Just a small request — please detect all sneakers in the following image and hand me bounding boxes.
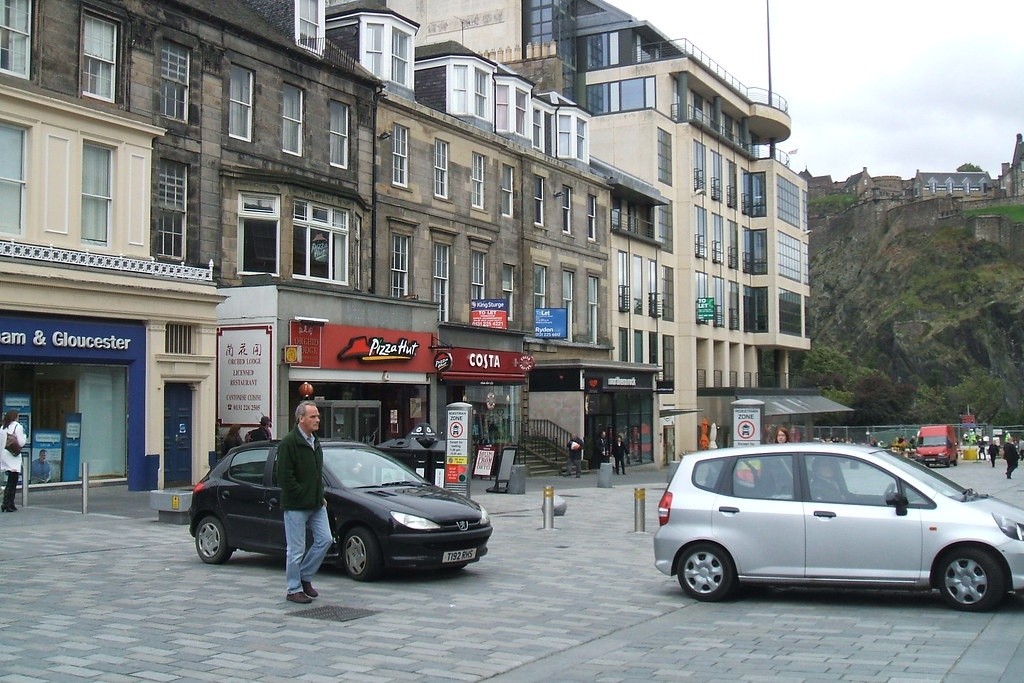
[300,579,317,597]
[286,591,312,604]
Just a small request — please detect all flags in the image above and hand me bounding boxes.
[788,149,798,154]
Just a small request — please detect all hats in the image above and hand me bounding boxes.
[259,416,269,425]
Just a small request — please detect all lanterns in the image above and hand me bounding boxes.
[299,382,314,398]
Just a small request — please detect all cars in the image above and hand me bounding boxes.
[652,443,1024,612]
[185,438,494,582]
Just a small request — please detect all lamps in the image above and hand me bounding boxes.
[552,191,565,197]
[379,131,391,140]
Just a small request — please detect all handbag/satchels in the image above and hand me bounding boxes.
[5,422,22,456]
[571,441,579,449]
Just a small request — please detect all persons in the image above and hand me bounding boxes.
[1003,437,1020,479]
[1012,438,1024,461]
[775,427,792,443]
[260,416,272,440]
[31,449,51,483]
[223,424,242,458]
[0,410,27,512]
[909,436,916,448]
[562,434,584,478]
[988,440,999,468]
[611,435,630,475]
[276,401,333,604]
[978,439,986,460]
[596,431,609,456]
[961,428,977,445]
[1004,430,1011,442]
[820,436,852,445]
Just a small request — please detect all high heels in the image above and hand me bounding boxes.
[1,503,14,512]
[10,503,18,511]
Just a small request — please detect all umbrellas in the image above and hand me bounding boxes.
[699,418,718,450]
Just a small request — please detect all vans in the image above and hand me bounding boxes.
[914,424,957,466]
